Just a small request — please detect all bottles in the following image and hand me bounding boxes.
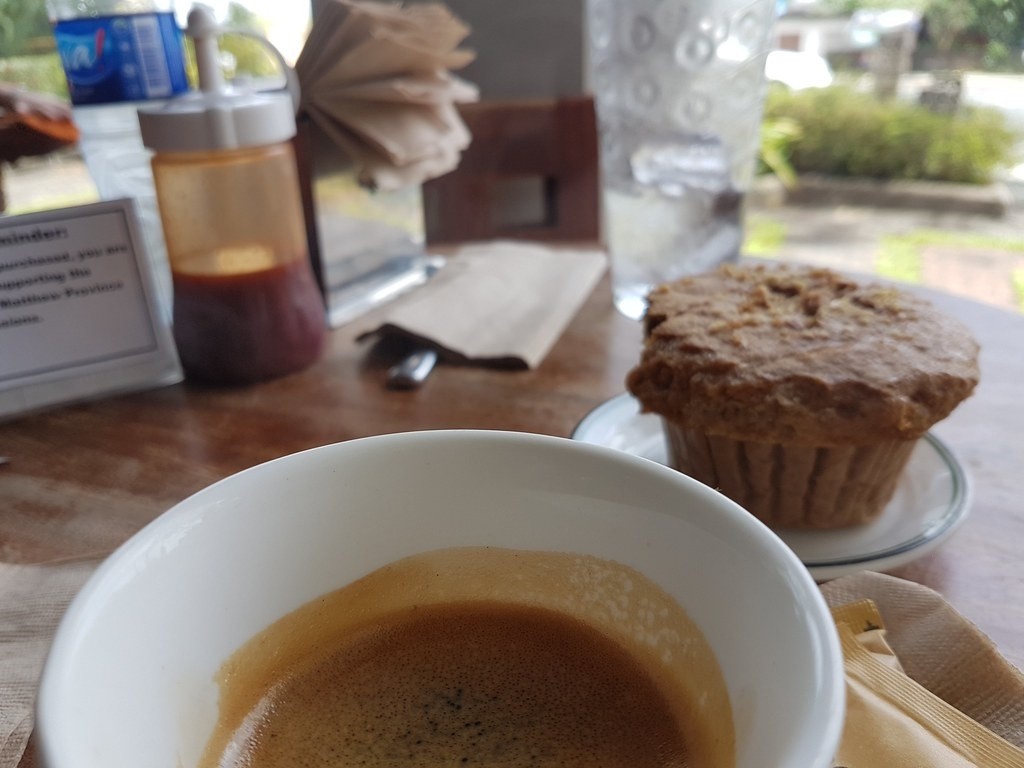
[42,0,194,329]
[136,5,329,387]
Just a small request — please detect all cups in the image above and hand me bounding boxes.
[582,0,777,320]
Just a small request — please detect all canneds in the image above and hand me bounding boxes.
[134,90,324,384]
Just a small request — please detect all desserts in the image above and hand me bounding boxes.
[626,263,983,530]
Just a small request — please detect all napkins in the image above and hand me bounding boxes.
[353,243,608,370]
[290,0,482,188]
[818,571,1024,751]
[0,560,104,768]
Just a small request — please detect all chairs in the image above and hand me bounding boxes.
[428,96,604,246]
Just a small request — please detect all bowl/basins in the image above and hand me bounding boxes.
[32,426,846,768]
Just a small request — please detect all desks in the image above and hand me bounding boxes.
[0,214,1024,768]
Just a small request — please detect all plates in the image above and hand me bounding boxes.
[570,390,971,581]
